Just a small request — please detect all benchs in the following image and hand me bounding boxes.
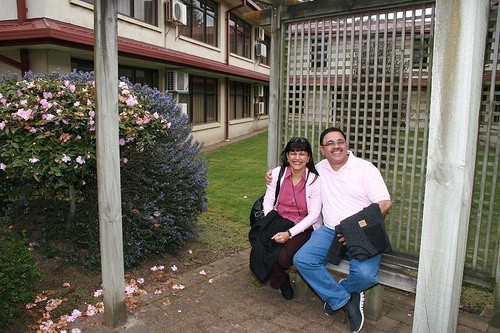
[296,251,419,321]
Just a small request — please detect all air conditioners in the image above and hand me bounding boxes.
[165,0,188,26]
[166,70,189,94]
[256,43,266,58]
[254,102,264,114]
[175,103,187,115]
[255,26,264,41]
[255,84,264,97]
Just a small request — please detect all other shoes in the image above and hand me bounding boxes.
[280,273,294,300]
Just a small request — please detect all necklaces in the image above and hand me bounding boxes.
[291,172,305,203]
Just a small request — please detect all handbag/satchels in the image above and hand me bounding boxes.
[250,195,264,227]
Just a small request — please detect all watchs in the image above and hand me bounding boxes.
[287,230,292,239]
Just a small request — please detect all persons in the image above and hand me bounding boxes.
[262,137,322,300]
[265,127,394,333]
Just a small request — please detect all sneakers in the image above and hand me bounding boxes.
[343,292,365,333]
[324,278,346,315]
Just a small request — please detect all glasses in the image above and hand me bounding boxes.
[290,137,308,144]
[323,140,346,147]
